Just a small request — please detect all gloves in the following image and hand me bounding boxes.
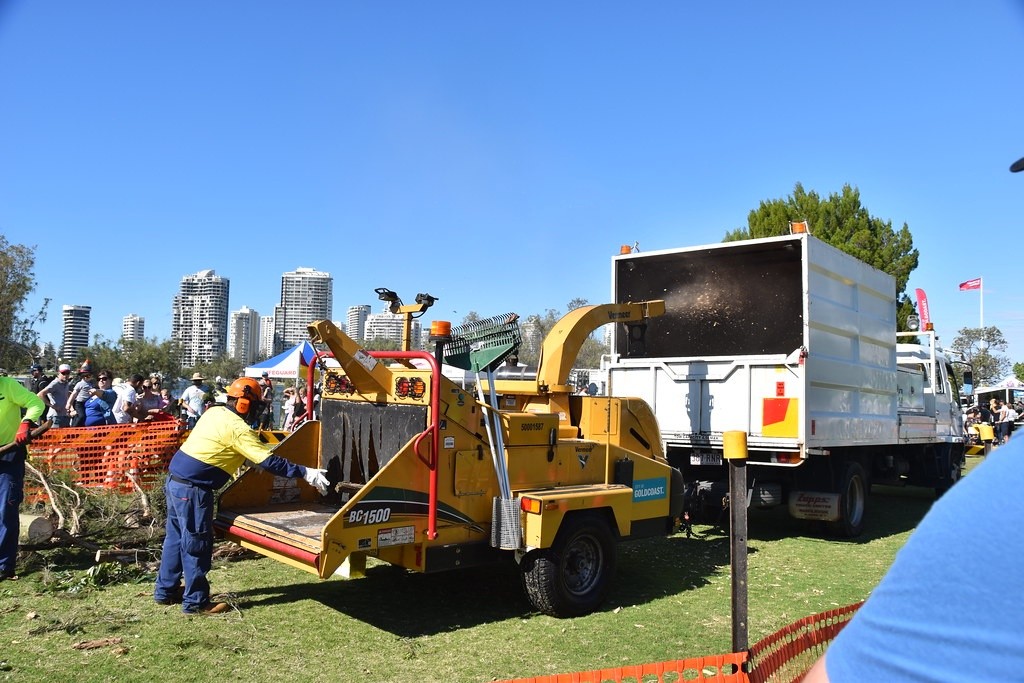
[304,466,331,497]
[14,421,30,448]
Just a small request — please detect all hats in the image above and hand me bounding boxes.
[58,364,70,373]
[189,373,207,380]
[262,372,268,376]
[28,363,43,372]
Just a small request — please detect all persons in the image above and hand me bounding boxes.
[0,359,322,493]
[963,398,1024,445]
[153,376,331,612]
[0,376,45,581]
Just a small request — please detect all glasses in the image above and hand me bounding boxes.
[142,385,152,389]
[153,382,160,385]
[61,371,70,375]
[97,377,108,381]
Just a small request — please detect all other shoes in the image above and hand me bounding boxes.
[195,602,230,614]
[0,569,15,582]
[155,597,181,605]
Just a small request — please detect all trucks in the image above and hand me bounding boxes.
[598,218,976,537]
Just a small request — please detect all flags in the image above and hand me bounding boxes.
[959,277,981,291]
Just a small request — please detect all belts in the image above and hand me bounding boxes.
[167,471,191,485]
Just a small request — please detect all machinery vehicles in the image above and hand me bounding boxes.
[214,299,688,623]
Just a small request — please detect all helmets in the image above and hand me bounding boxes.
[227,377,262,414]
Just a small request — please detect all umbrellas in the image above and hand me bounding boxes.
[244,340,323,387]
